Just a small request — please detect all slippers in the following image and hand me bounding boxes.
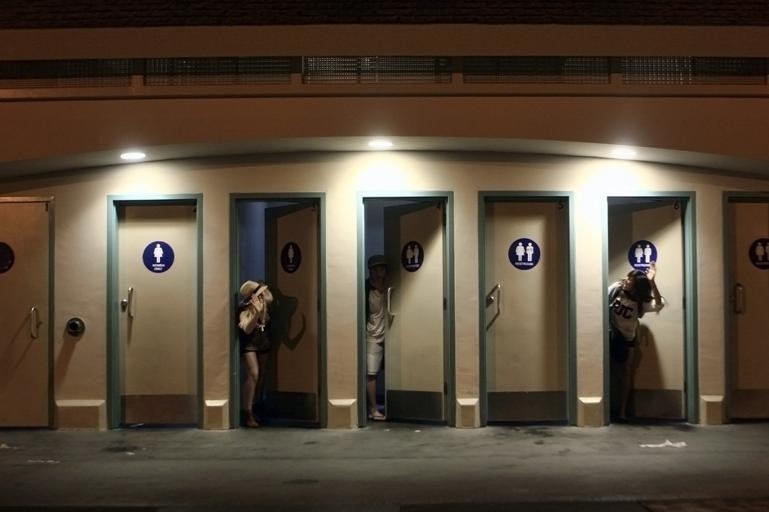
[368,413,387,420]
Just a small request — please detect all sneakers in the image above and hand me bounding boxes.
[241,410,259,427]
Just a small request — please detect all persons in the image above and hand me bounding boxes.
[236,279,273,428]
[363,253,388,423]
[608,260,666,421]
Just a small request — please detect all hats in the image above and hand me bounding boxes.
[239,279,268,305]
[365,255,392,269]
